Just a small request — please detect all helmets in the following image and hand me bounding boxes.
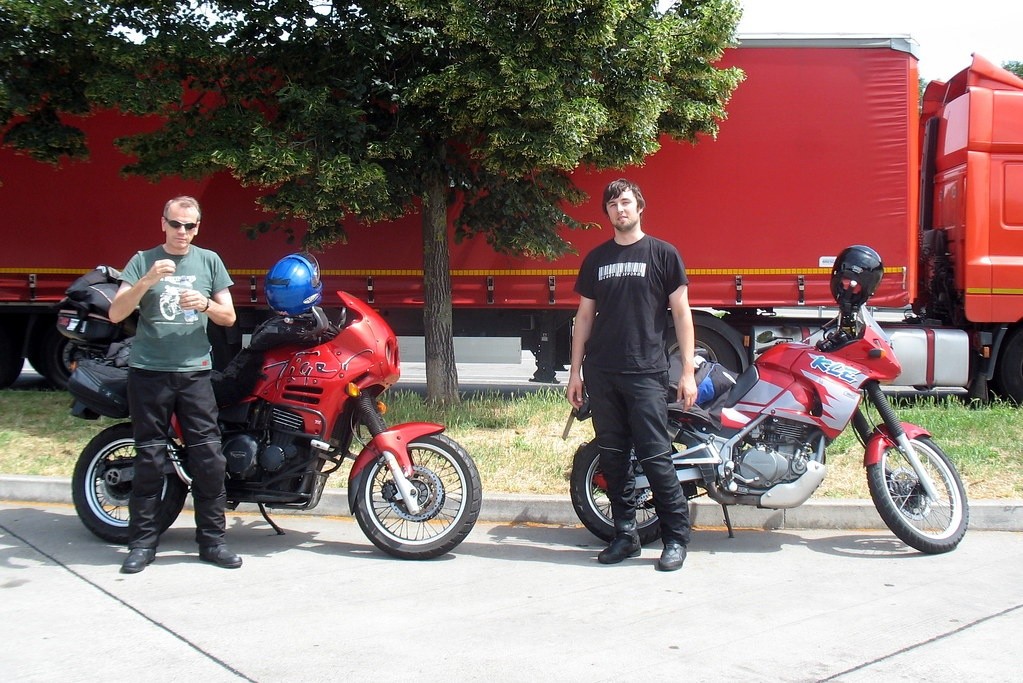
[263,250,324,318]
[831,245,884,314]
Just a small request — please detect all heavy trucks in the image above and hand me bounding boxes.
[0,31,1023,414]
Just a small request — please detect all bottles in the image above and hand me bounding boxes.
[178,275,199,323]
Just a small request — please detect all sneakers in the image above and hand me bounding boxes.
[123,548,156,573]
[199,545,243,568]
[658,539,687,571]
[598,531,641,564]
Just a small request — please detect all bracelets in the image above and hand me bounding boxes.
[200,298,210,313]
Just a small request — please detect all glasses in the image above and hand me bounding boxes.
[163,216,198,229]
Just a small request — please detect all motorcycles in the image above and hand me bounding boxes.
[562,298,969,550]
[63,287,487,560]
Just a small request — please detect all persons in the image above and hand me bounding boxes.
[107,195,245,576]
[567,177,701,569]
